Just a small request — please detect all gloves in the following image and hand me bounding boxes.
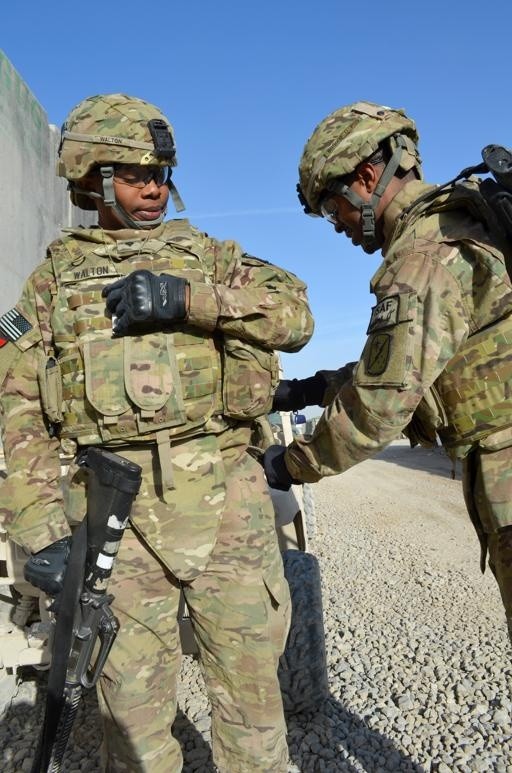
[23,537,73,595]
[259,444,303,491]
[269,374,324,414]
[102,270,186,333]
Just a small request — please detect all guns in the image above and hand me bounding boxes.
[481,144,512,231]
[17,446,142,769]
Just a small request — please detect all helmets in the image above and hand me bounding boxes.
[299,101,419,217]
[59,94,178,179]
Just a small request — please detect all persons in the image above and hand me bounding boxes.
[0,93,316,773]
[264,98,512,647]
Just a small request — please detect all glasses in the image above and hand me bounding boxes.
[321,156,385,224]
[112,164,168,187]
